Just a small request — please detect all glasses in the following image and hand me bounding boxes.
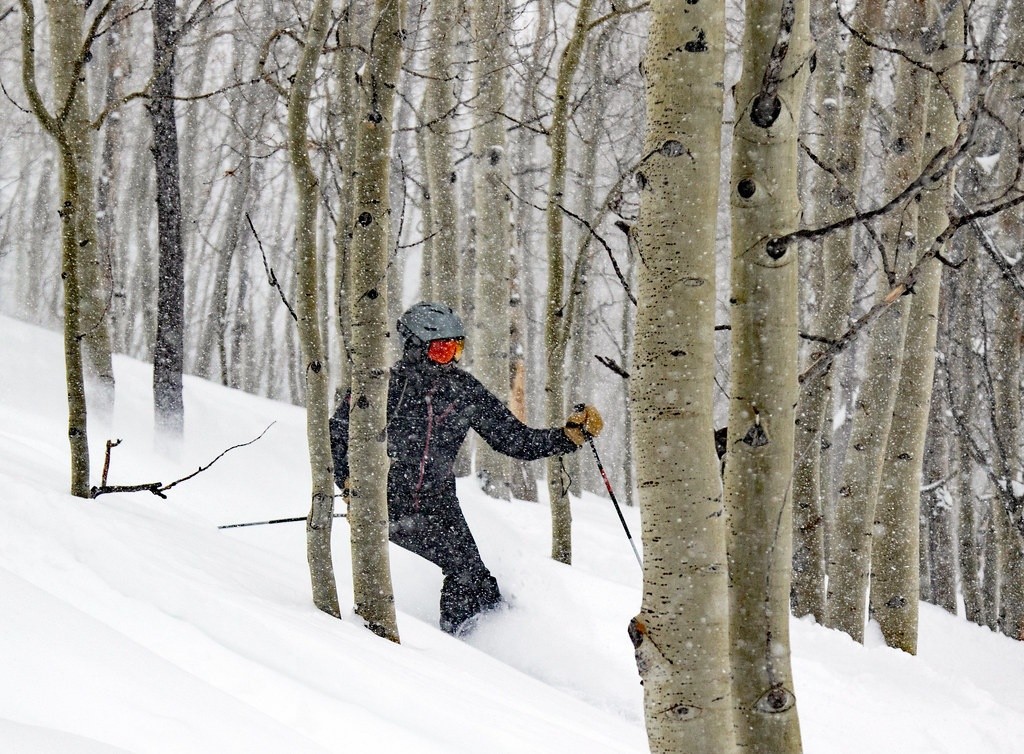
[425,335,466,364]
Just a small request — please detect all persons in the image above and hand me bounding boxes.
[328,301,604,639]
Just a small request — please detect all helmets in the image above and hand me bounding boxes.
[397,301,464,374]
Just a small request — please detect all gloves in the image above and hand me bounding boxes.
[564,404,602,446]
[342,478,350,505]
